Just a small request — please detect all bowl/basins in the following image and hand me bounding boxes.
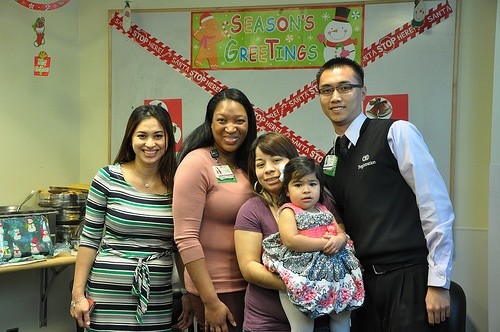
[37,194,88,223]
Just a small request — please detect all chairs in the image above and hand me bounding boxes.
[427,280,466,332]
[68,279,86,332]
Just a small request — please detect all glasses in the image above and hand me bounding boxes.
[317,83,363,97]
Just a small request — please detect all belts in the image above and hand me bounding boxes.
[361,257,429,275]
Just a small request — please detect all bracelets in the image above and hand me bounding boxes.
[180,288,188,294]
[71,295,85,308]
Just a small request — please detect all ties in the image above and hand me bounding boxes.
[336,133,350,161]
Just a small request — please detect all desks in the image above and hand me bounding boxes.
[0,252,79,331]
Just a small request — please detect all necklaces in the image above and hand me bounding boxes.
[135,162,160,188]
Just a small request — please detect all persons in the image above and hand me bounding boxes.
[69,105,194,332]
[315,56,455,332]
[171,87,257,332]
[215,167,221,174]
[233,131,346,332]
[261,156,365,332]
